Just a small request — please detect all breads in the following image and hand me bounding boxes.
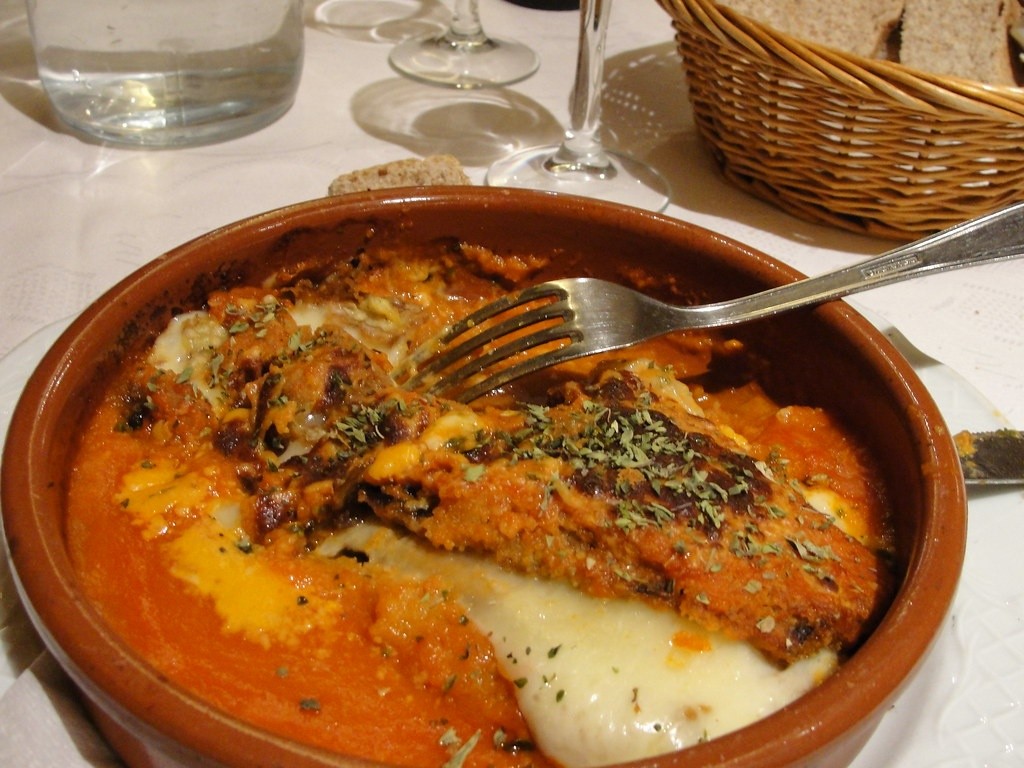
[715,0,1022,88]
[329,152,469,194]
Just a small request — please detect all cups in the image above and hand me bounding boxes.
[28,0,308,150]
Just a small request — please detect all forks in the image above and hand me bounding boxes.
[389,195,1024,405]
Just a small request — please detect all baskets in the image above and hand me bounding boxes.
[655,0,1024,241]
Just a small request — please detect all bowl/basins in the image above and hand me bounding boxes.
[2,183,968,768]
